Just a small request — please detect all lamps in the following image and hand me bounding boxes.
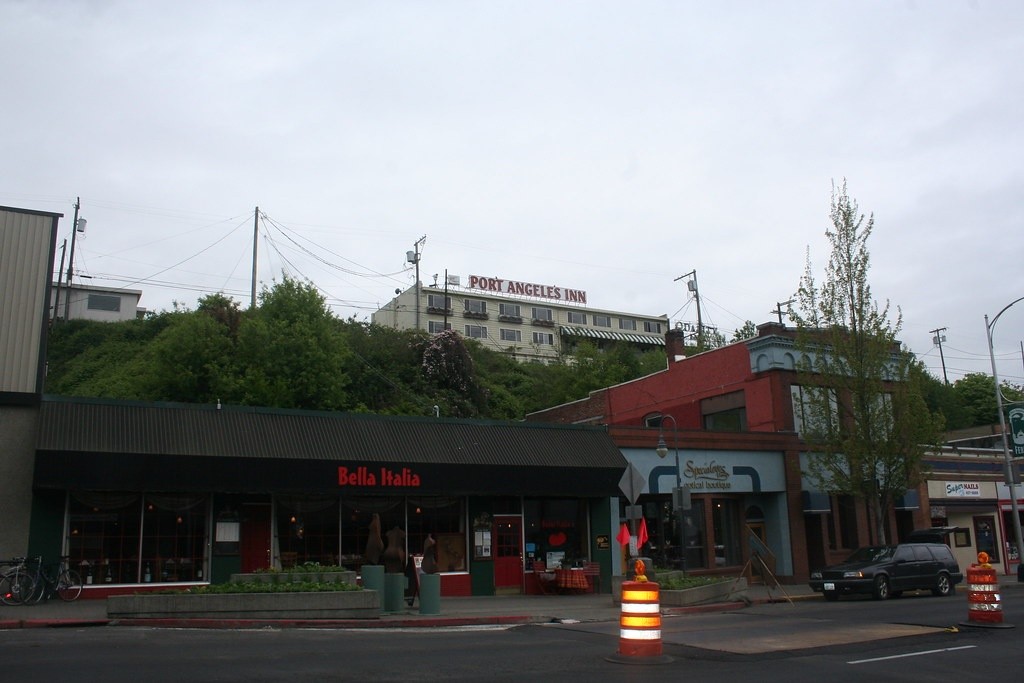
[217,399,221,409]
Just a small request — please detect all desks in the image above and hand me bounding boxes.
[556,569,589,595]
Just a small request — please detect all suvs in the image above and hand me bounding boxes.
[808,526,963,600]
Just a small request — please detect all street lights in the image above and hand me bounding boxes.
[656,414,686,578]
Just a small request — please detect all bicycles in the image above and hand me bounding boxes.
[0,554,83,606]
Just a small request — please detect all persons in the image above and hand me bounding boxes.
[80,552,111,576]
[365,513,383,555]
[384,526,406,560]
[423,534,436,574]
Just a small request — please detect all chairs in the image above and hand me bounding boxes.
[583,562,600,596]
[533,561,558,595]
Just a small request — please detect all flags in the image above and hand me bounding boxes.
[637,516,648,548]
[616,523,631,546]
